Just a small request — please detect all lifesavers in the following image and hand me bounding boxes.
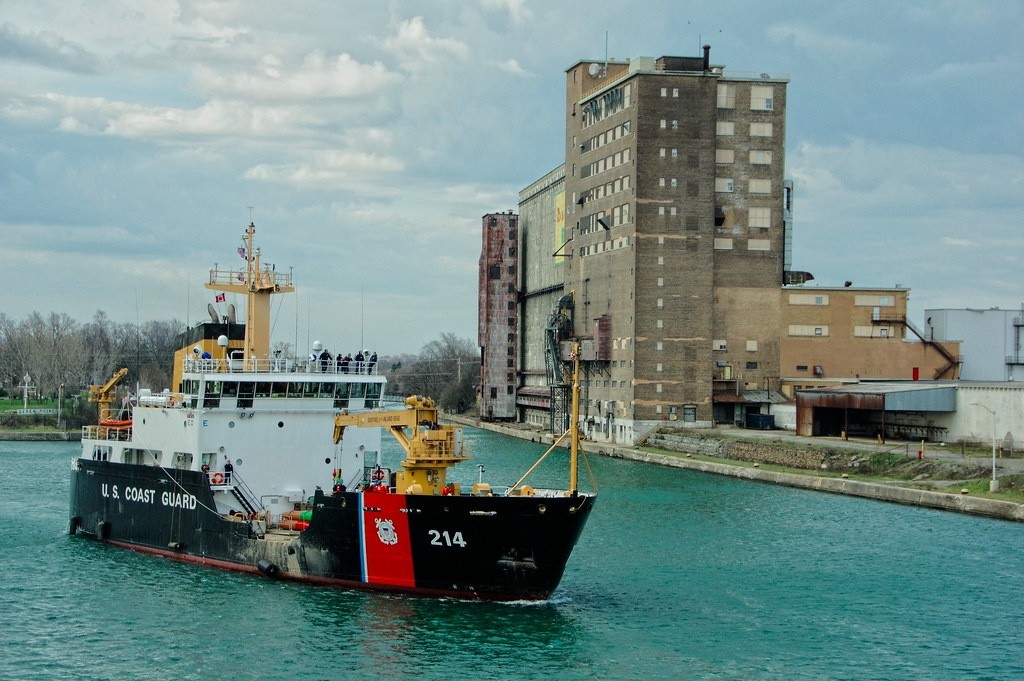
[373,469,384,481]
[167,396,175,406]
[212,473,223,484]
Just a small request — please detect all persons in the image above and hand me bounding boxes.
[201,462,211,484]
[223,460,234,485]
[368,352,377,375]
[341,353,352,374]
[336,354,343,374]
[319,349,333,373]
[355,351,365,374]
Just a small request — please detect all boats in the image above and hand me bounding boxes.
[67,221,601,605]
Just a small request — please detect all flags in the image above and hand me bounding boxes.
[216,293,226,302]
